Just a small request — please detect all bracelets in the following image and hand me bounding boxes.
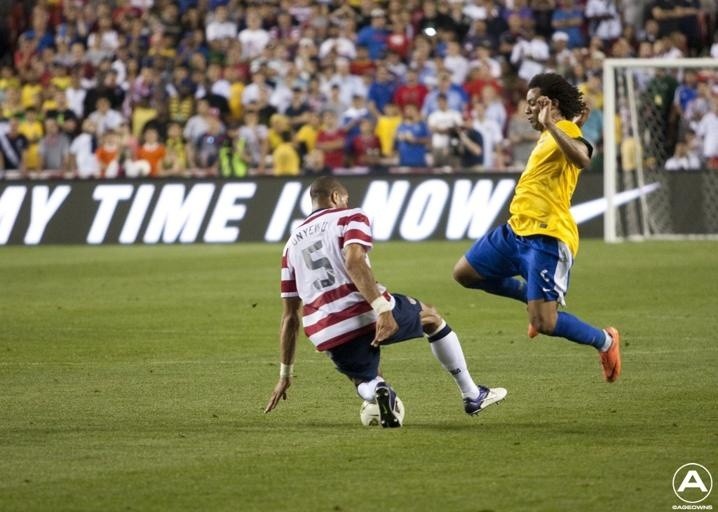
[279,362,294,379]
[369,295,393,316]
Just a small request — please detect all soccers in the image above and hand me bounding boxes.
[359,394,405,428]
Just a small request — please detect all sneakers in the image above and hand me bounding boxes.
[463,385,507,416]
[600,327,621,382]
[376,381,402,428]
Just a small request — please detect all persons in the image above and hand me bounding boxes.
[263,176,508,430]
[451,73,622,384]
